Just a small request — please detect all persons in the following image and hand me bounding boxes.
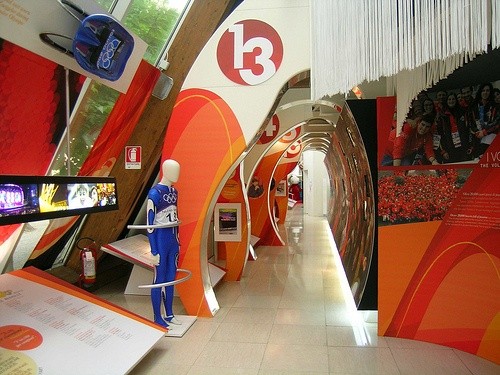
[68,184,95,210]
[381,80,500,166]
[146,159,182,330]
[247,177,264,198]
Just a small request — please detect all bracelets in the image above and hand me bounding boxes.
[431,158,436,162]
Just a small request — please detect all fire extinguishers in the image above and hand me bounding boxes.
[75,236,98,293]
